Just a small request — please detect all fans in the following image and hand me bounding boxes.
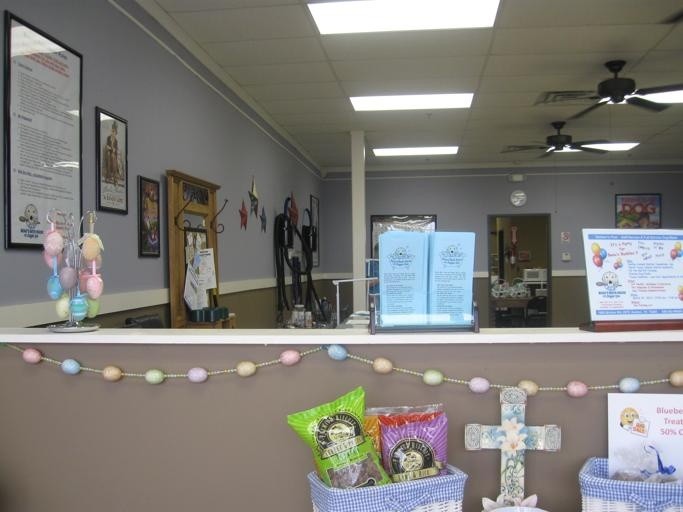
[509,121,610,159]
[567,60,683,121]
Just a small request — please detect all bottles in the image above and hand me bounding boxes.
[291,305,313,328]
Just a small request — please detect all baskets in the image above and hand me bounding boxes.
[577,456,682,512]
[306,462,469,511]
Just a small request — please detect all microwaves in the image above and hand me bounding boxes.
[523,268,548,282]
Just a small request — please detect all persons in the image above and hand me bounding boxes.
[105,121,120,188]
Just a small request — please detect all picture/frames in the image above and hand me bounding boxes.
[3,10,84,251]
[616,193,662,229]
[370,214,437,260]
[95,106,130,214]
[310,194,320,268]
[136,174,161,258]
